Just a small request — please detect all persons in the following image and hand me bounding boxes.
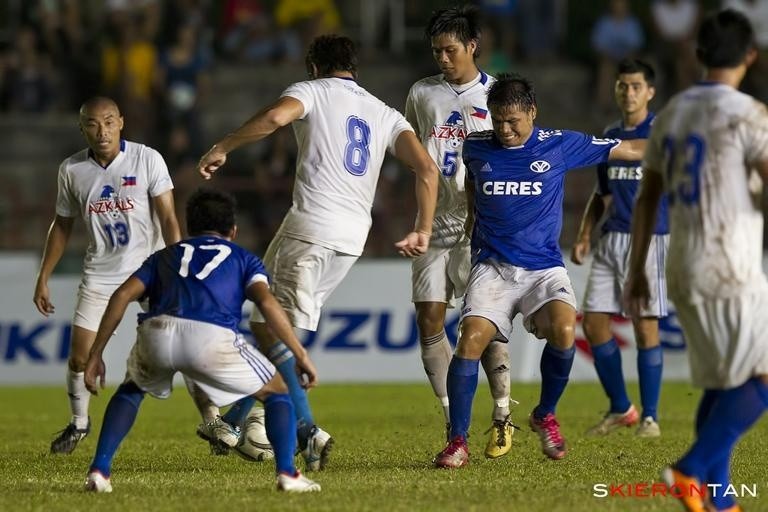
[428,67,652,474]
[0,1,768,253]
[570,55,672,442]
[82,181,321,493]
[194,31,439,475]
[401,7,522,460]
[30,94,232,456]
[614,6,768,512]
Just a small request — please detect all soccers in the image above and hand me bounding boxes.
[232,407,274,462]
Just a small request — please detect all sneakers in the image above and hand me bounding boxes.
[584,404,639,440]
[50,416,92,454]
[704,481,743,512]
[196,415,243,449]
[84,467,112,493]
[209,438,229,455]
[301,425,334,472]
[662,463,707,512]
[433,405,566,467]
[634,417,660,437]
[275,470,322,493]
